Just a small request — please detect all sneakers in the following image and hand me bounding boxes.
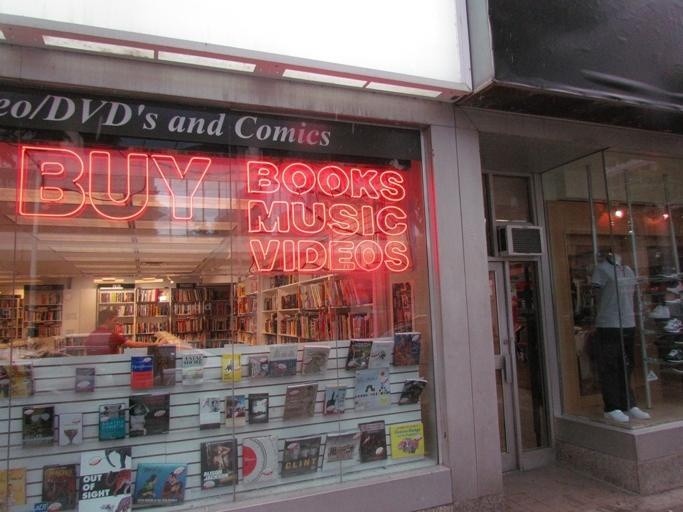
[602,409,630,423]
[623,406,650,419]
[647,272,683,373]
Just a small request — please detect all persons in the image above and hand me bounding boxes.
[591,251,651,425]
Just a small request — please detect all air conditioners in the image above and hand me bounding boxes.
[497,223,544,257]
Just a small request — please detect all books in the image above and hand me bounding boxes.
[95,283,228,348]
[182,346,356,490]
[345,332,429,463]
[229,265,377,346]
[1,345,187,511]
[0,290,63,341]
